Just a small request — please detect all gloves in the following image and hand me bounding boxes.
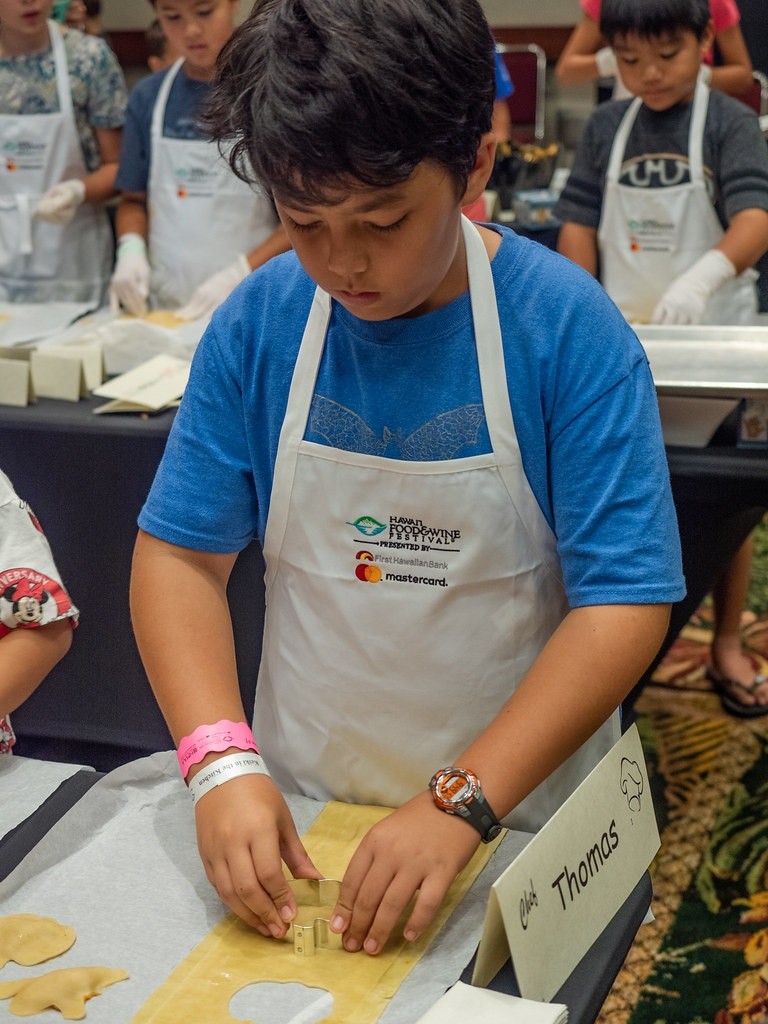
[36,180,85,226]
[653,249,737,326]
[181,253,253,319]
[109,233,150,316]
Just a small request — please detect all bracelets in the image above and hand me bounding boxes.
[177,719,264,786]
[189,752,272,809]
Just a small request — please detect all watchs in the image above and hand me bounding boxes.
[429,767,502,845]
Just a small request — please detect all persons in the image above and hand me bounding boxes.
[131,0,688,956]
[556,0,754,101]
[51,0,174,70]
[0,470,78,757]
[553,1,768,718]
[0,0,130,303]
[114,0,292,316]
[491,55,515,143]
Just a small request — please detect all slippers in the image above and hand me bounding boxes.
[706,666,768,714]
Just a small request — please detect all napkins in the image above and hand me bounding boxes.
[414,980,569,1024]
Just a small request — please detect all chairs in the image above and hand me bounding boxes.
[494,43,547,145]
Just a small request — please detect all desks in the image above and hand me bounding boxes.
[0,300,768,772]
[494,187,600,284]
[0,751,653,1024]
